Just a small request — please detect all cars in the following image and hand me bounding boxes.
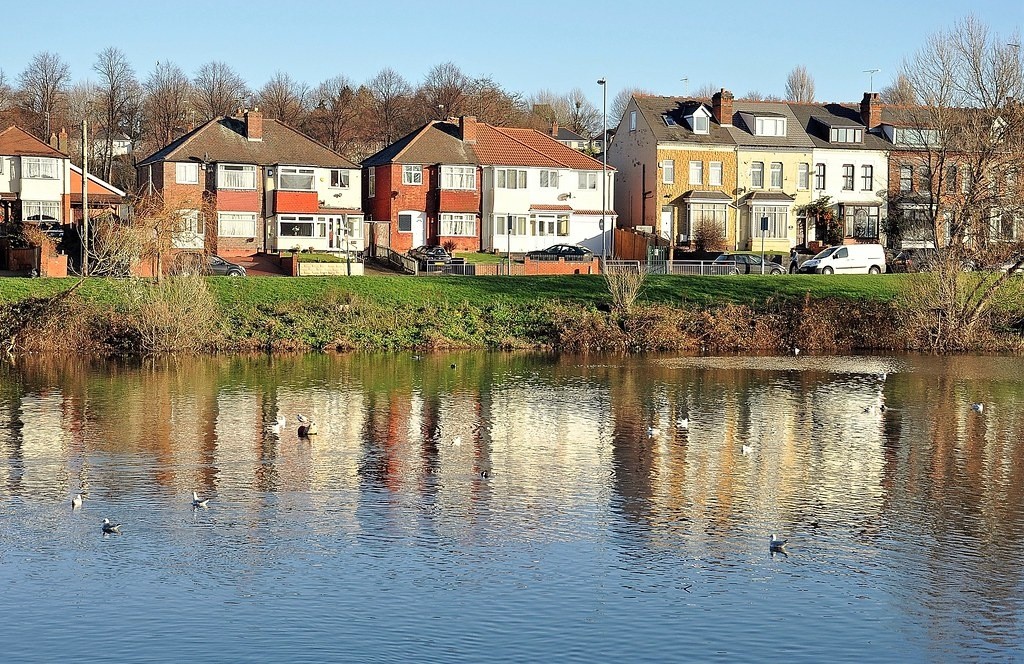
[523,244,594,263]
[711,253,787,276]
[409,245,452,271]
[984,250,1024,272]
[890,249,975,274]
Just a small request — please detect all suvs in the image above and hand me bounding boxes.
[168,250,247,277]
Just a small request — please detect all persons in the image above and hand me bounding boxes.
[789,247,799,274]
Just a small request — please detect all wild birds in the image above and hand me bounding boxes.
[859,403,886,413]
[101,517,122,533]
[191,491,211,509]
[768,531,790,547]
[293,411,317,442]
[450,363,458,369]
[646,416,689,437]
[479,466,494,478]
[740,443,753,455]
[793,346,798,352]
[973,400,983,412]
[451,437,461,446]
[8,419,24,425]
[410,354,423,360]
[87,411,101,422]
[72,493,84,509]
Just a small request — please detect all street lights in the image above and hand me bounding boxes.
[597,76,607,261]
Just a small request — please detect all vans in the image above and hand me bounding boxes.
[798,244,886,275]
